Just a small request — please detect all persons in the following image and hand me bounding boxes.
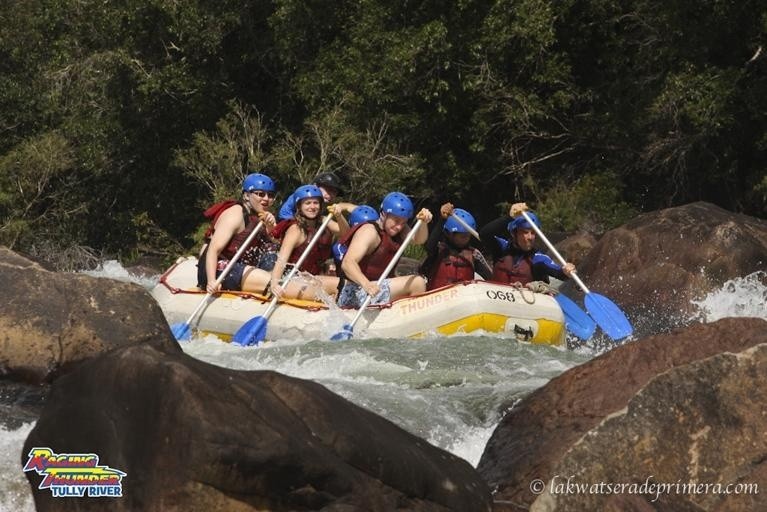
[267,183,348,306]
[332,201,380,275]
[478,203,578,295]
[337,189,432,307]
[421,200,492,294]
[196,170,280,294]
[278,171,342,222]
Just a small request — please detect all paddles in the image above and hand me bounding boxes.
[516,202,632,342]
[230,210,328,343]
[167,222,266,341]
[447,208,597,341]
[331,215,423,336]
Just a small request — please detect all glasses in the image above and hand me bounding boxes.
[251,190,275,198]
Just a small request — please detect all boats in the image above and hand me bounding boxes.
[153,254,567,352]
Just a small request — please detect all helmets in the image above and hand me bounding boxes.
[508,212,539,231]
[313,174,344,194]
[383,193,413,218]
[243,174,275,192]
[348,206,378,224]
[294,185,324,204]
[444,209,476,233]
